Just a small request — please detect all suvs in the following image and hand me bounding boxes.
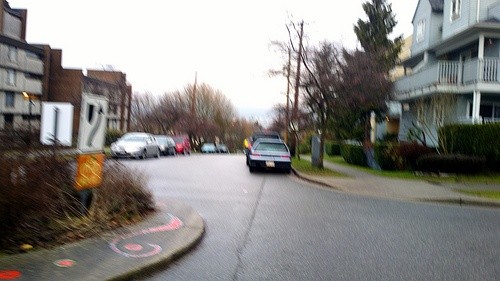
[246,134,280,167]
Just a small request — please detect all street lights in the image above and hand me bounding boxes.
[21,90,35,130]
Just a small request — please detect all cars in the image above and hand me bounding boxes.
[110,132,160,159]
[248,138,292,174]
[154,135,175,156]
[201,142,216,154]
[170,136,191,155]
[218,144,228,153]
